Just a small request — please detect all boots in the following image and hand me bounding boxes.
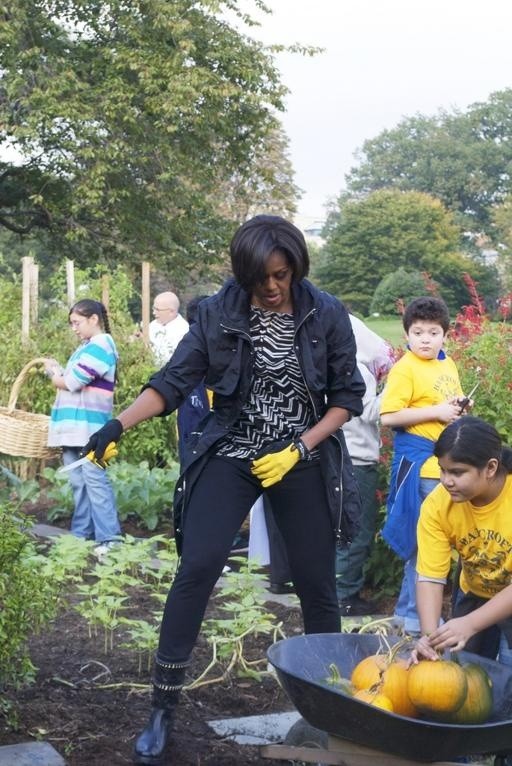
[133,652,191,759]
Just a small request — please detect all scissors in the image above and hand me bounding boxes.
[456,380,483,416]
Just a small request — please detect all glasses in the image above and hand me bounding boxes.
[152,306,170,313]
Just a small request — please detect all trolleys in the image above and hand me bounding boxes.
[252,631,512,766]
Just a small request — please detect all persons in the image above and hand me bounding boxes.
[41,301,124,544]
[378,297,475,638]
[405,417,512,671]
[81,218,366,766]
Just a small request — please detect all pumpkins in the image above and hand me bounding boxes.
[323,654,493,724]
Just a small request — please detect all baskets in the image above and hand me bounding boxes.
[0,355,67,461]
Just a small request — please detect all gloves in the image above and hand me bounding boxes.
[249,437,311,488]
[78,418,123,469]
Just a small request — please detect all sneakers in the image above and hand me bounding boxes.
[94,540,380,617]
